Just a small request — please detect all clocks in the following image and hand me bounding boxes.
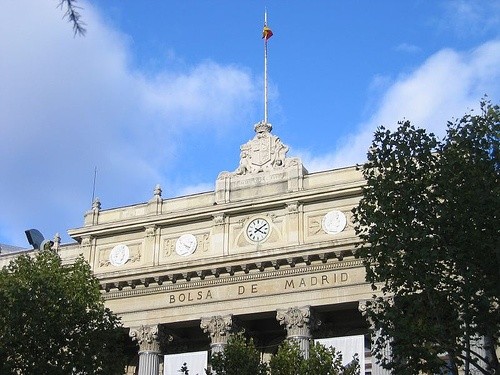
[246,218,269,242]
